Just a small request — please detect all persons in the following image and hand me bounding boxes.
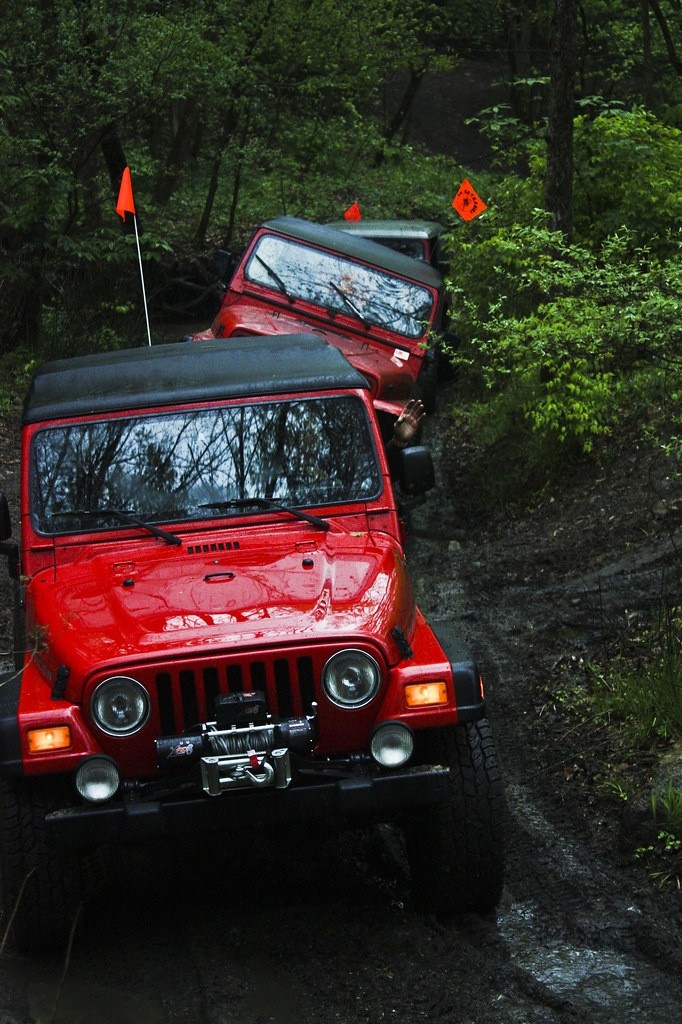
[385,398,427,472]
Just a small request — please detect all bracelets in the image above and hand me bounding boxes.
[391,438,408,447]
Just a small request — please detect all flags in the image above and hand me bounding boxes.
[451,179,488,222]
[343,201,362,223]
[116,167,135,223]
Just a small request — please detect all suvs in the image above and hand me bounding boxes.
[323,220,451,280]
[191,216,446,453]
[0,331,506,966]
